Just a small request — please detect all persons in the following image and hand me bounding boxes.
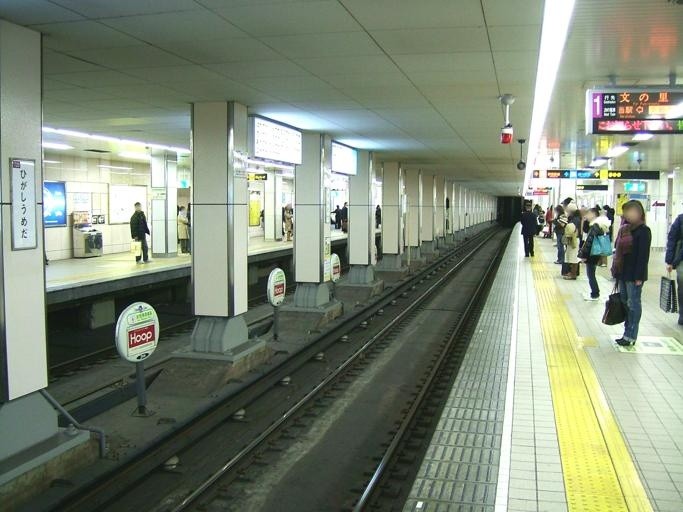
[610,199,651,347]
[282,207,293,242]
[177,202,191,253]
[130,202,153,264]
[665,213,683,325]
[521,198,615,302]
[375,205,381,228]
[341,202,348,231]
[330,205,342,229]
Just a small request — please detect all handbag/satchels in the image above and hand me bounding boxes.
[590,225,612,256]
[602,277,625,325]
[130,238,142,256]
[659,270,677,313]
[542,225,550,235]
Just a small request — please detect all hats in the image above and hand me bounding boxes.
[524,203,531,208]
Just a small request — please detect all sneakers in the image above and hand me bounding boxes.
[553,260,576,279]
[582,291,598,300]
[615,338,635,346]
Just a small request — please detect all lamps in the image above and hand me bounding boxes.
[517,139,526,171]
[501,93,516,145]
